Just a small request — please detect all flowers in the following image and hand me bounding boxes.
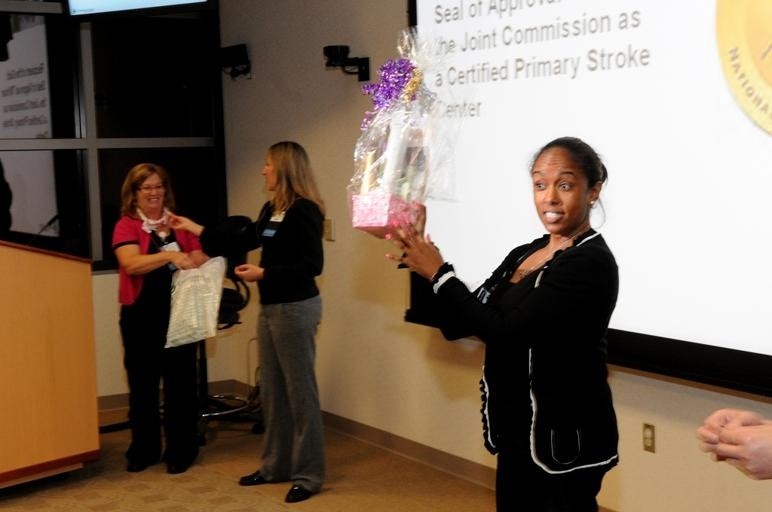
[355,55,427,133]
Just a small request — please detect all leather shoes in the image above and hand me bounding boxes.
[285,484,319,503]
[238,469,287,486]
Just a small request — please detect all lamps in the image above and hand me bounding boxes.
[218,42,254,84]
[323,44,372,83]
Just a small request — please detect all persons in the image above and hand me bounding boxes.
[385,135,623,512]
[165,140,326,507]
[112,160,212,476]
[691,407,771,484]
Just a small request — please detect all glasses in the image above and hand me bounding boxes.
[135,185,163,192]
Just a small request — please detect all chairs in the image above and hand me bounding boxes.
[160,214,254,447]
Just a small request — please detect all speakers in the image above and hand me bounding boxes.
[220,43,250,78]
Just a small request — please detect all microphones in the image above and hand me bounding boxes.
[38,214,59,235]
